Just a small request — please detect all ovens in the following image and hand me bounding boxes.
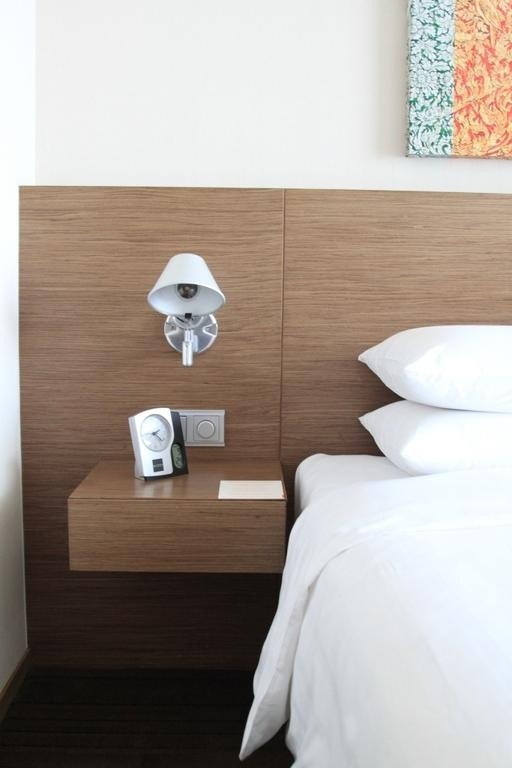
[273,453,512,767]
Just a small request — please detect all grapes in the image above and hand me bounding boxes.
[68,498,290,575]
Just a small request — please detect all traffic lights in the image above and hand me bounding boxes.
[353,326,512,477]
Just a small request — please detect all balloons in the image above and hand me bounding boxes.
[127,407,189,481]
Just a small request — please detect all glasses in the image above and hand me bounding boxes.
[146,252,227,368]
